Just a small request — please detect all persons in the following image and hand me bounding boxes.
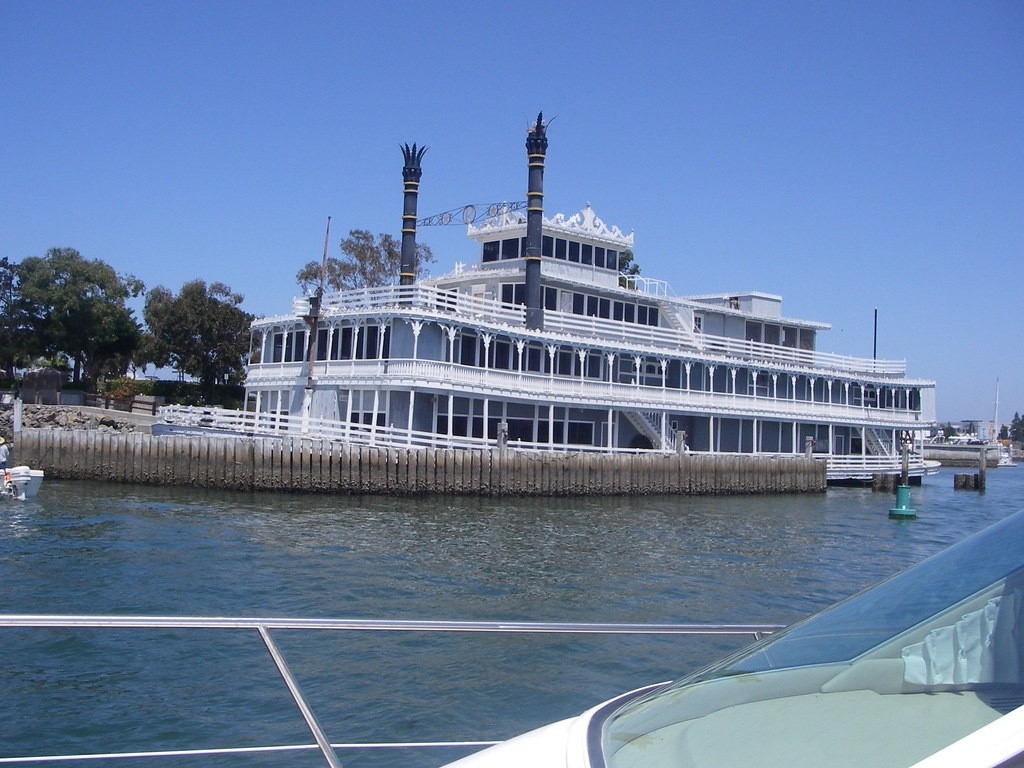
[0,437,9,469]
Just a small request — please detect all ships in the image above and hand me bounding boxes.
[161,106,948,487]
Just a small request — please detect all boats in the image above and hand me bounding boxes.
[998,442,1019,468]
[0,465,46,499]
